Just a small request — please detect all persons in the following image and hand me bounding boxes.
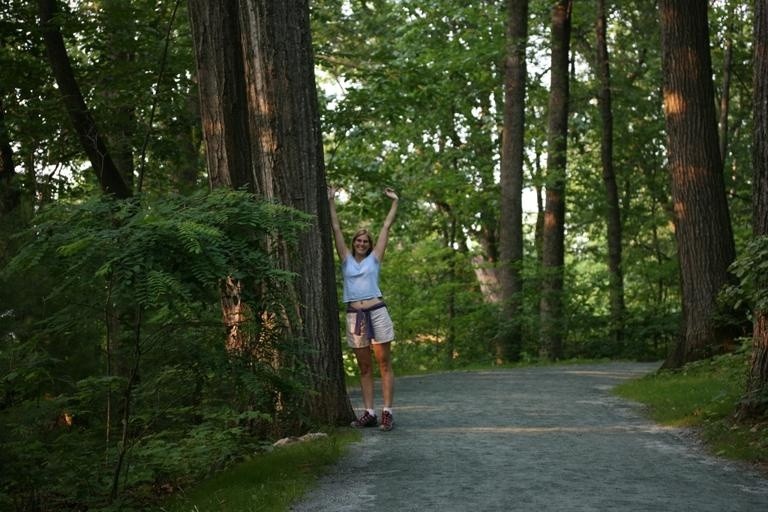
[327,184,401,431]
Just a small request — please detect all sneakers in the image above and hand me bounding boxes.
[351,410,379,429]
[380,411,394,432]
